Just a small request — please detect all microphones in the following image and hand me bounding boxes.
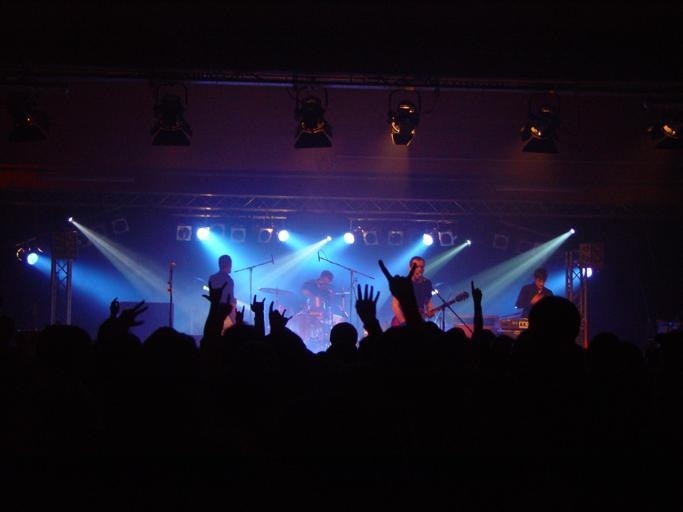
[318,251,320,261]
[272,256,274,264]
[168,257,176,267]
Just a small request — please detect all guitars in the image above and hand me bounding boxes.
[391,292,469,327]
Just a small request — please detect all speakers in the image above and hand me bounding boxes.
[64,231,79,259]
[579,242,592,268]
[591,240,610,271]
[50,232,65,259]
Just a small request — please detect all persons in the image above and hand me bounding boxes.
[1,256,681,512]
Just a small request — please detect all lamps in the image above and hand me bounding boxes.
[645,105,682,150]
[520,91,559,155]
[6,87,53,143]
[293,84,334,148]
[148,79,193,148]
[386,88,422,147]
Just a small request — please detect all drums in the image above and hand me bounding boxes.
[305,297,326,316]
[289,312,331,354]
[319,304,348,324]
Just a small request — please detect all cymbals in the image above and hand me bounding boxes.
[260,289,293,295]
